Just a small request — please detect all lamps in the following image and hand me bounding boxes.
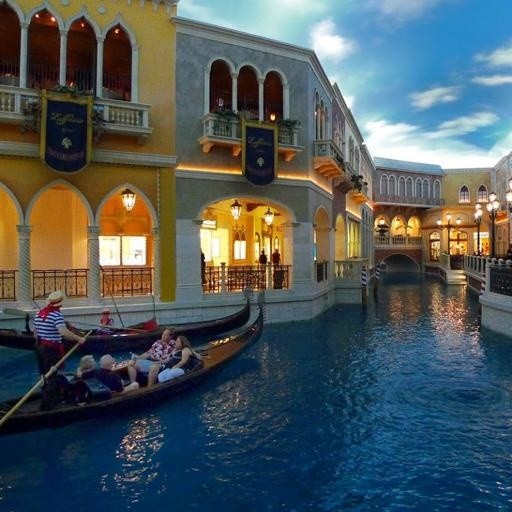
[215,96,225,113]
[262,206,275,226]
[230,197,243,221]
[120,188,137,214]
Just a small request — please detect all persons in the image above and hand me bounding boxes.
[259,251,267,273]
[80,355,97,373]
[95,308,115,336]
[127,327,202,386]
[77,355,139,397]
[32,290,86,393]
[507,244,512,259]
[158,335,196,383]
[273,249,281,268]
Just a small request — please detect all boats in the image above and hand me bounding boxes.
[0,302,265,447]
[0,294,251,355]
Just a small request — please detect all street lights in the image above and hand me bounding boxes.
[504,176,512,258]
[473,201,483,255]
[437,212,463,256]
[485,191,501,257]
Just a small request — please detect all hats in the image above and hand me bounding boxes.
[45,290,67,307]
